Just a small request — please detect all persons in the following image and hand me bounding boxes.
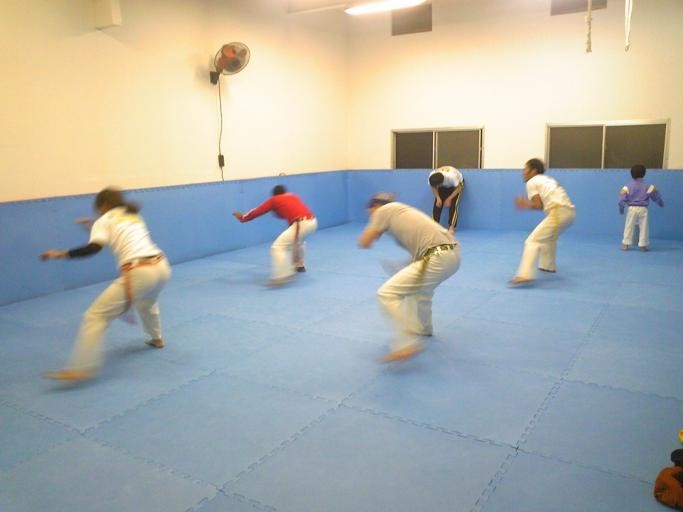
[427,165,464,234]
[232,186,318,287]
[358,192,461,363]
[618,165,665,250]
[507,158,576,286]
[38,187,172,384]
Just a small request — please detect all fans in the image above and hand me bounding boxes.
[209,40,251,85]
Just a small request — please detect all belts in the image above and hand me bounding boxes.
[416,244,455,281]
[119,255,163,314]
[290,216,314,260]
